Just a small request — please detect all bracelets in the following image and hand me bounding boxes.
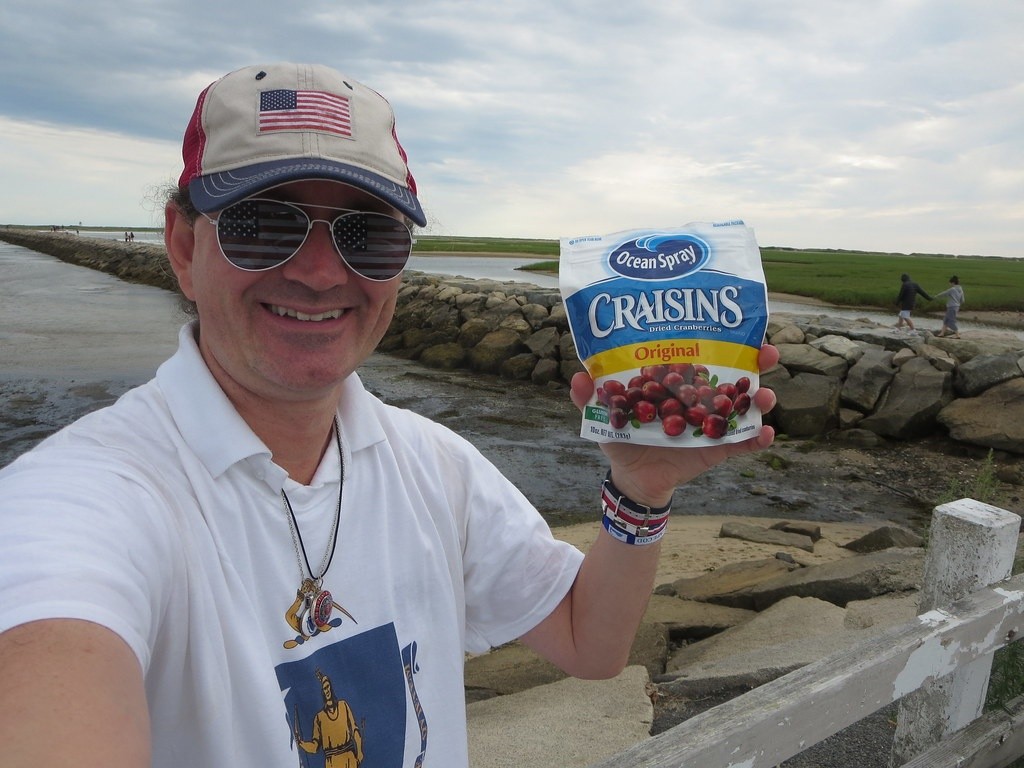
[601,464,674,546]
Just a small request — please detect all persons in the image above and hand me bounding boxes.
[2,58,783,768]
[893,274,933,332]
[48,224,81,239]
[123,230,136,244]
[934,275,965,339]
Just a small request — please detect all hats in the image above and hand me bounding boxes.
[178,62,428,229]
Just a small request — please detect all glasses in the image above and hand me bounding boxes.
[195,197,417,282]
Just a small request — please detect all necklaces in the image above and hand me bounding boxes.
[281,408,344,637]
